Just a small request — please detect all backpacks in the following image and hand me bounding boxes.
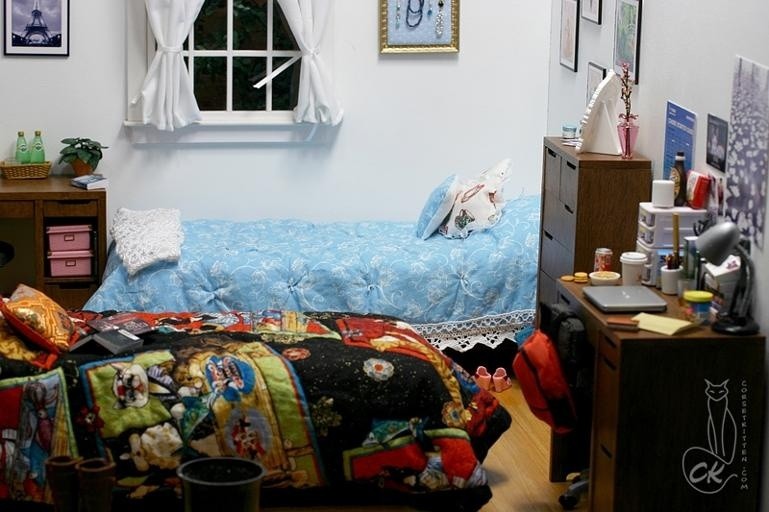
[515,301,583,435]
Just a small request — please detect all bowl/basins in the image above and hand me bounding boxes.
[588,270,620,286]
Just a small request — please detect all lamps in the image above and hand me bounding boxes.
[695,221,760,337]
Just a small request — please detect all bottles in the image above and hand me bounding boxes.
[594,248,613,272]
[15,131,30,163]
[681,290,713,326]
[668,151,686,207]
[30,131,45,163]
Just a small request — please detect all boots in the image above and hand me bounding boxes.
[46,457,81,512]
[77,460,116,512]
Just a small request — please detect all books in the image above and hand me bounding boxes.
[70,173,107,189]
[69,324,90,351]
[703,255,743,319]
[94,328,144,355]
[87,310,149,334]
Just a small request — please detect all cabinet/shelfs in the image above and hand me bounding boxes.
[550,273,611,305]
[554,274,769,512]
[535,137,652,332]
[0,174,107,310]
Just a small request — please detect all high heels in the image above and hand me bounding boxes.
[493,368,511,392]
[471,367,491,390]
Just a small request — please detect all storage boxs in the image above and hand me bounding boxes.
[46,225,92,251]
[47,251,94,277]
[637,202,707,249]
[636,240,685,286]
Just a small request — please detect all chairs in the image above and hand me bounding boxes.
[538,302,591,510]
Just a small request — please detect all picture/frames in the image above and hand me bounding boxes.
[581,0,602,25]
[4,0,70,56]
[586,62,606,107]
[378,0,461,54]
[559,0,580,72]
[612,0,643,85]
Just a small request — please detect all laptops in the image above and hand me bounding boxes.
[582,285,667,313]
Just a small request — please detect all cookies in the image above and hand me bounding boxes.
[560,271,588,283]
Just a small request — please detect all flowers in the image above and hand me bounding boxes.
[618,63,636,156]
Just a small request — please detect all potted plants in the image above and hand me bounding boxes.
[58,137,109,177]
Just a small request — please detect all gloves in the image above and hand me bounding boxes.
[1,159,51,179]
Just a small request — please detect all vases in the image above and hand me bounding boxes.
[617,122,640,159]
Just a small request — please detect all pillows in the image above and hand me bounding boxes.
[416,173,458,240]
[438,158,513,239]
[1,283,76,357]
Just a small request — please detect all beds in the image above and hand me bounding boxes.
[0,311,512,512]
[81,194,543,353]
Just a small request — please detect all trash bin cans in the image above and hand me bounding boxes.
[176,456,267,512]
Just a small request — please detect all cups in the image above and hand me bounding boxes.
[619,251,648,286]
[661,265,684,295]
[677,277,698,307]
